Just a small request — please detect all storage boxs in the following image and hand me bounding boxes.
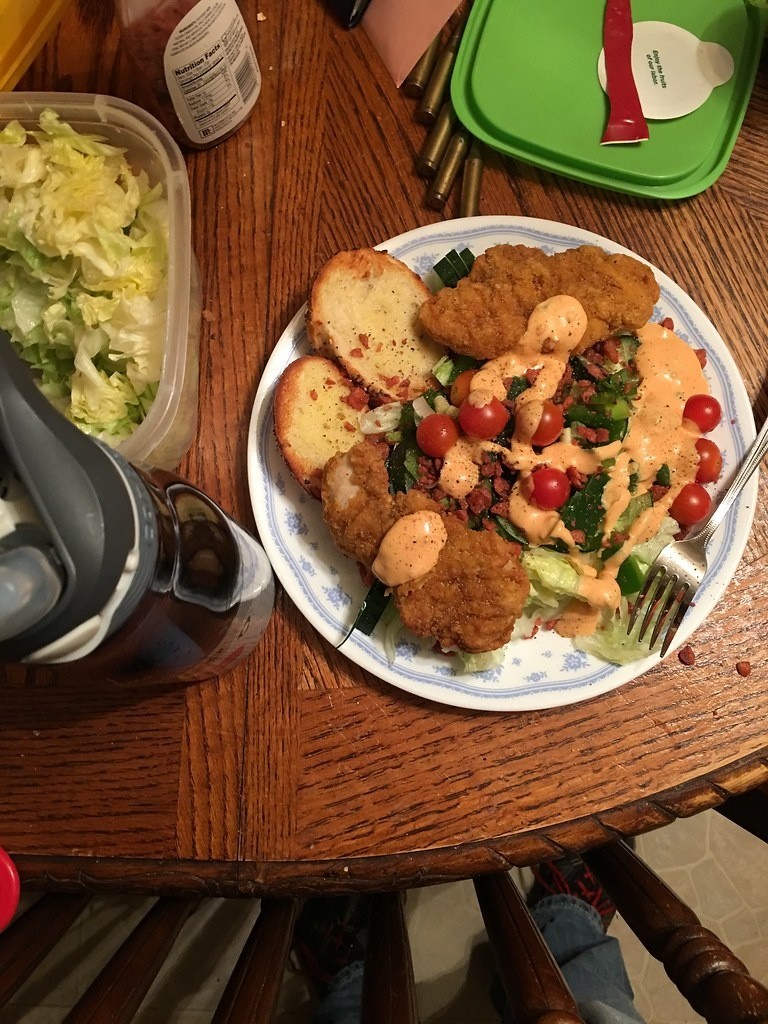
[0,87,200,471]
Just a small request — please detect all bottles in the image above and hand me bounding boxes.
[0,334,276,691]
[117,0,261,150]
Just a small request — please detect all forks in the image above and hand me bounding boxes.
[626,417,768,658]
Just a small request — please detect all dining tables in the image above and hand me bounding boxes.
[0,0,767,897]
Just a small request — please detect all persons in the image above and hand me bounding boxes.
[291,833,646,1023]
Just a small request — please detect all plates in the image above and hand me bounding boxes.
[246,216,759,711]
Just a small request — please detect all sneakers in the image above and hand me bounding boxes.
[527,834,638,936]
[299,887,409,995]
